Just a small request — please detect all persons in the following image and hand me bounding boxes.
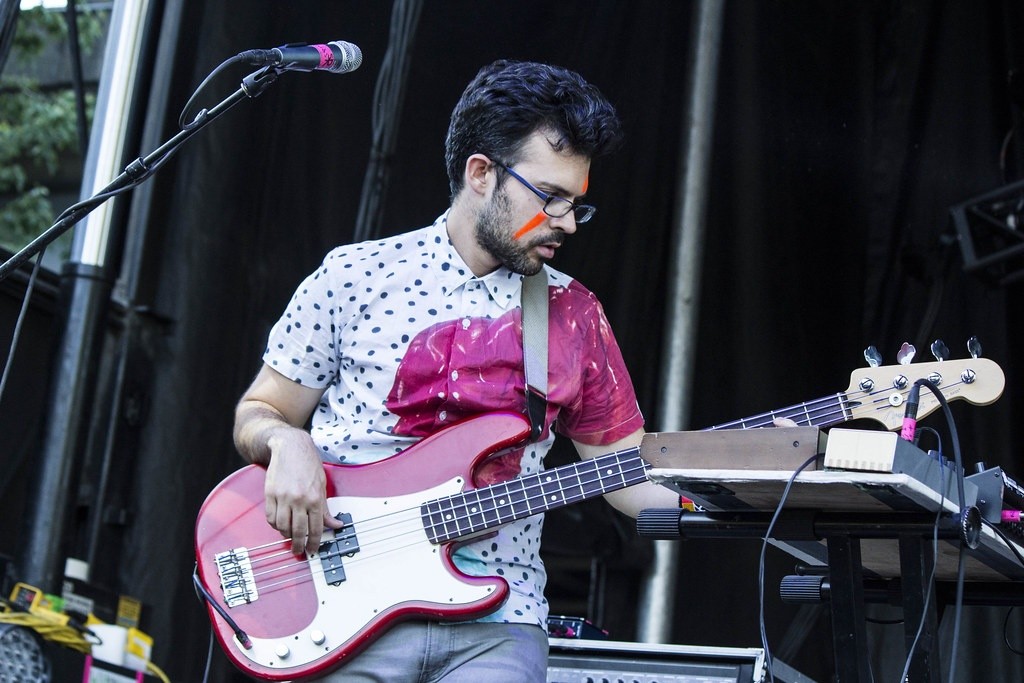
[231,59,802,682]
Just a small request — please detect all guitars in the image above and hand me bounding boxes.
[193,339,1006,683]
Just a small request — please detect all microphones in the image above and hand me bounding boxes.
[241,41,362,74]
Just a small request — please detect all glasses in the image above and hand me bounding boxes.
[491,159,596,223]
